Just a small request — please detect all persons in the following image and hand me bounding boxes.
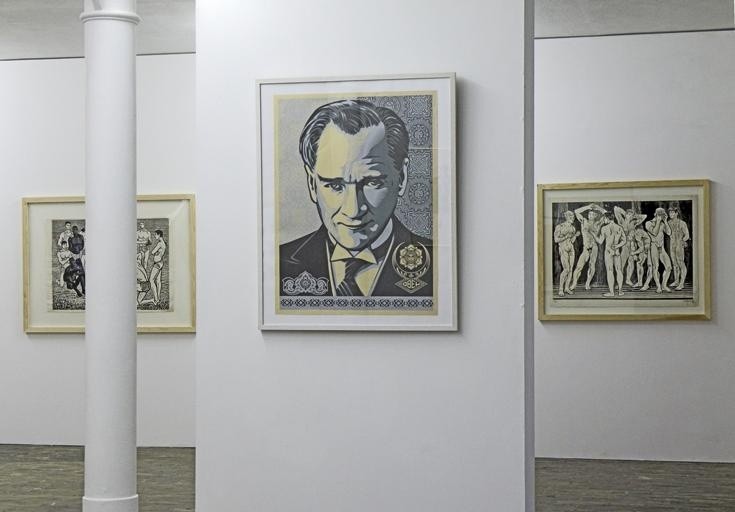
[280,100,433,296]
[553,202,691,299]
[56,221,167,305]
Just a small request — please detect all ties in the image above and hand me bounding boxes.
[336,258,372,296]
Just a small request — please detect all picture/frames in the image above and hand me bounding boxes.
[535,178,713,321]
[256,71,461,334]
[21,193,196,335]
